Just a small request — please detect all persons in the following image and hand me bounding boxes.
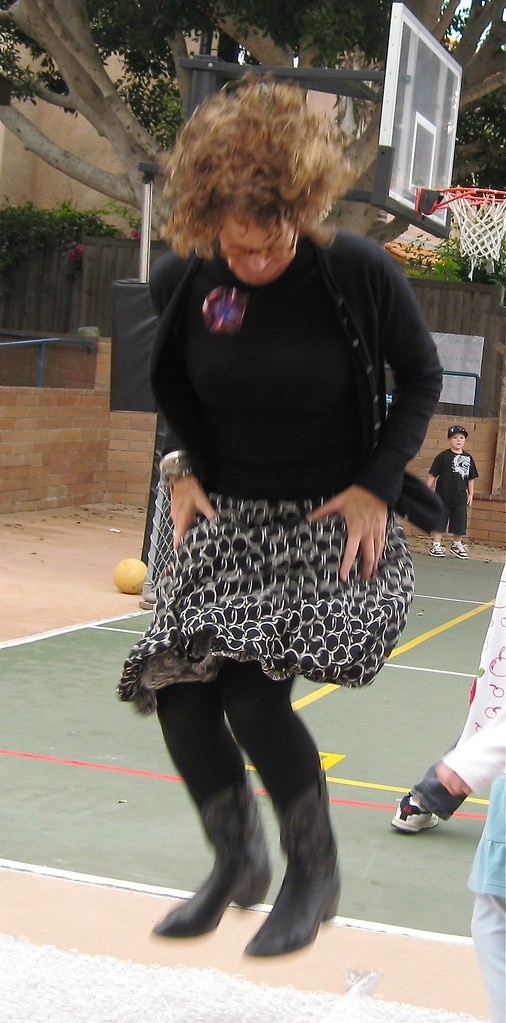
[390,707,506,1022]
[116,89,444,956]
[427,426,479,559]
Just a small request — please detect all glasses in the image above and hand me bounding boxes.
[214,225,297,260]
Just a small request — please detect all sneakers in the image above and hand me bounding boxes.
[429,542,446,557]
[391,791,439,833]
[449,541,469,559]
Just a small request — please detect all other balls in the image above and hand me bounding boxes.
[113,557,148,595]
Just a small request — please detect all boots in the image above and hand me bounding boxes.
[245,769,341,956]
[153,774,273,939]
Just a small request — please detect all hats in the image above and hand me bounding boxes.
[448,425,468,439]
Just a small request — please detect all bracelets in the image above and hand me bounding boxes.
[160,449,191,488]
